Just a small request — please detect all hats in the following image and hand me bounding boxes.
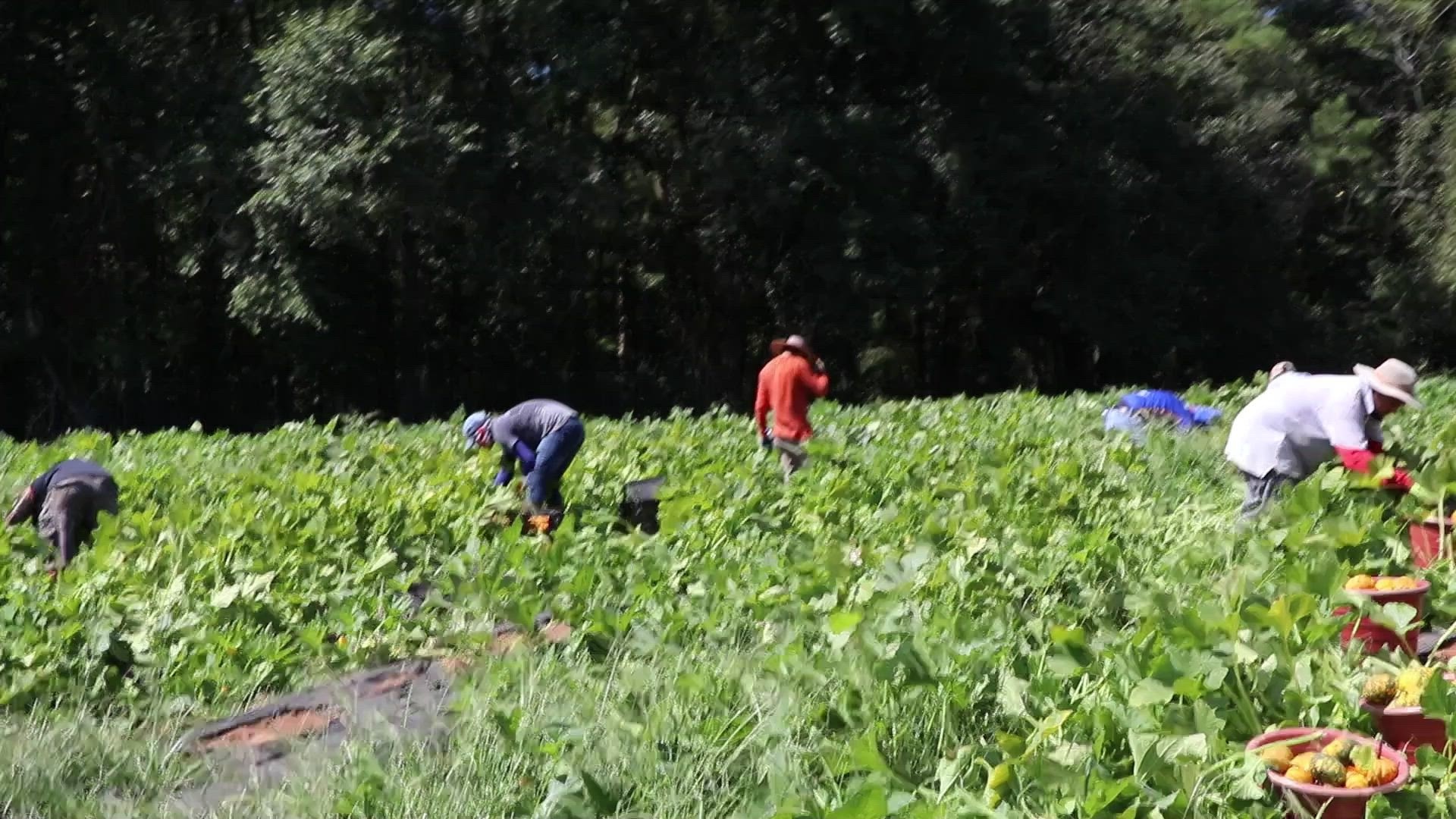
[461,409,490,455]
[1353,358,1423,411]
[771,334,814,362]
[1270,361,1296,380]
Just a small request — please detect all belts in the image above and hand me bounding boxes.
[1114,403,1142,417]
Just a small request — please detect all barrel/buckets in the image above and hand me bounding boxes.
[1335,577,1431,656]
[1247,728,1408,819]
[1360,675,1456,774]
[620,473,669,534]
[1407,513,1453,565]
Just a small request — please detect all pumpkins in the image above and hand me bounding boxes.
[1362,665,1438,707]
[1262,730,1398,788]
[1345,573,1417,590]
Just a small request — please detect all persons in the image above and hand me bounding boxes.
[1408,507,1456,569]
[5,458,118,570]
[1270,361,1296,382]
[462,398,585,537]
[1104,389,1219,434]
[1223,357,1437,520]
[755,335,829,484]
